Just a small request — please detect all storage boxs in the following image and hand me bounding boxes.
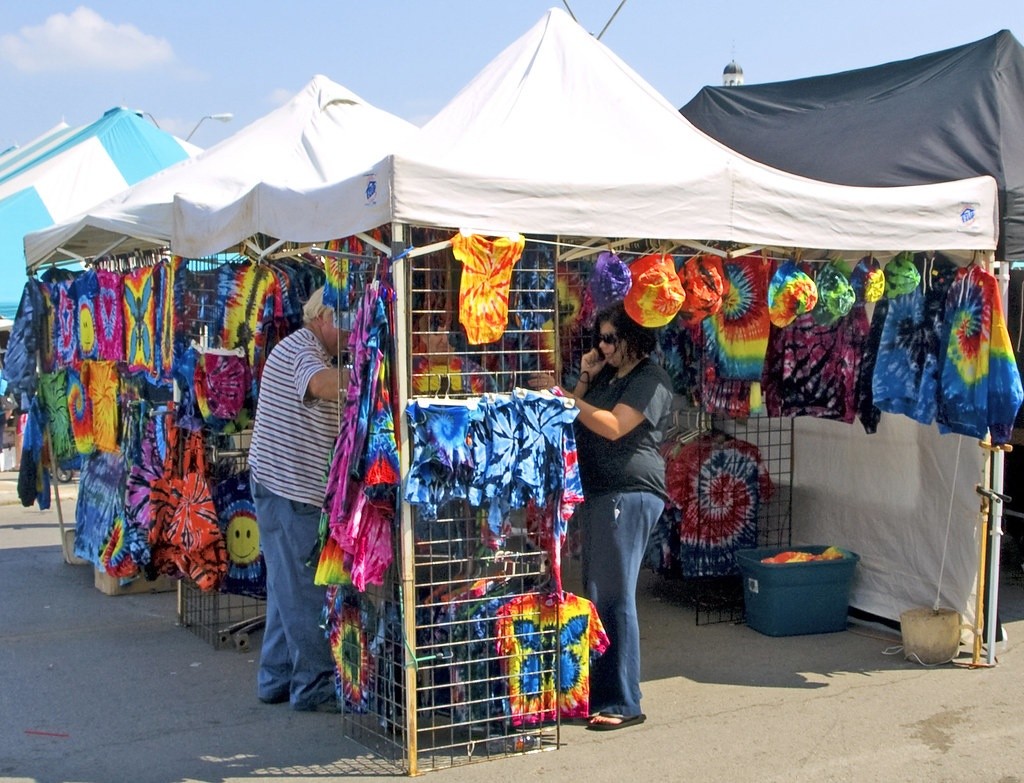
[733,545,861,638]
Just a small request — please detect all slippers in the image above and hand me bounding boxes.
[589,713,647,730]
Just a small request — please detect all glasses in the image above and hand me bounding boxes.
[597,334,617,343]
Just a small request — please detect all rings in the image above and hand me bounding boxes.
[544,381,549,387]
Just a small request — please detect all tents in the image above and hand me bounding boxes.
[0,6,1024,781]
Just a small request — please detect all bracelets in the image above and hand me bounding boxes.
[577,376,592,388]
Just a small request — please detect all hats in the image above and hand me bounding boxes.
[768,251,920,327]
[589,252,723,327]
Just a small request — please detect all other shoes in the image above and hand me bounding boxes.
[313,695,340,713]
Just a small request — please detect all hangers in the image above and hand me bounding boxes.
[964,249,977,268]
[454,547,556,599]
[665,408,712,444]
[407,371,574,411]
[450,548,562,592]
[225,232,310,273]
[99,245,168,273]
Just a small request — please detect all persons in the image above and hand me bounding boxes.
[0,359,28,472]
[542,300,675,730]
[414,307,499,394]
[246,281,371,714]
